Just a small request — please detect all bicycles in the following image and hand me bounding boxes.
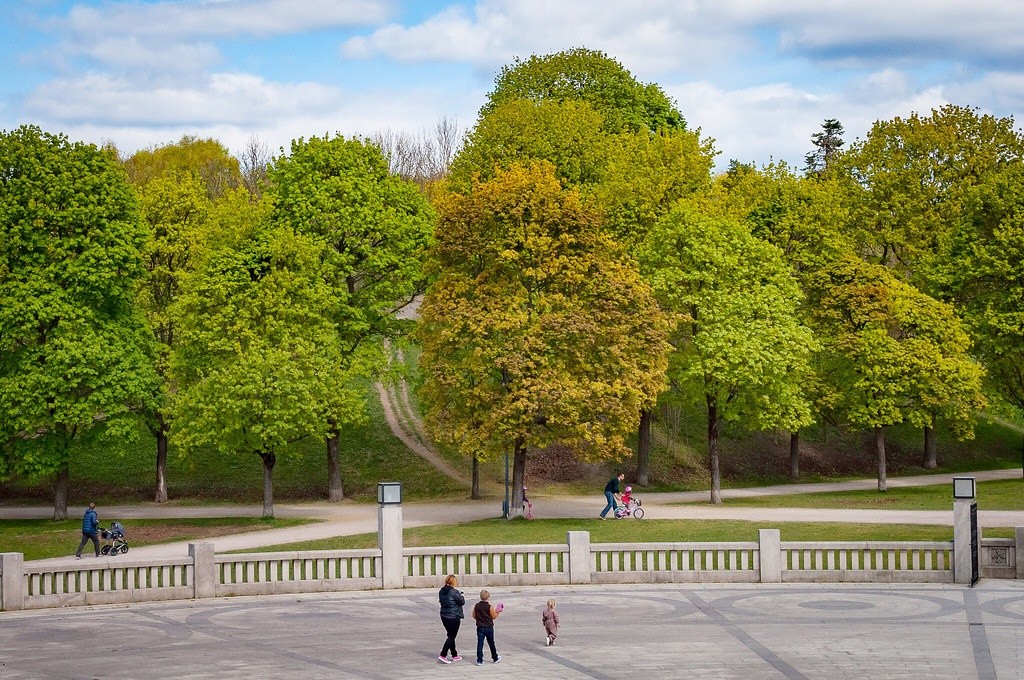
[614,497,644,520]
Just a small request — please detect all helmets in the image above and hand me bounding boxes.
[625,486,632,492]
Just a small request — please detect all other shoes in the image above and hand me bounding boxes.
[438,655,451,663]
[600,514,607,520]
[477,661,484,666]
[553,642,555,645]
[76,557,81,560]
[453,656,462,661]
[546,637,550,645]
[616,514,623,520]
[495,656,501,664]
[99,554,103,556]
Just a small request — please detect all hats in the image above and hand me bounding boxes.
[523,485,528,489]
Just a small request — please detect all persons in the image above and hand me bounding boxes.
[437,574,465,664]
[621,487,635,516]
[523,485,529,513]
[76,502,104,560]
[542,598,560,646]
[472,589,503,666]
[599,473,624,521]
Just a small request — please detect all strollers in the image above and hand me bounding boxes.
[98,522,128,556]
